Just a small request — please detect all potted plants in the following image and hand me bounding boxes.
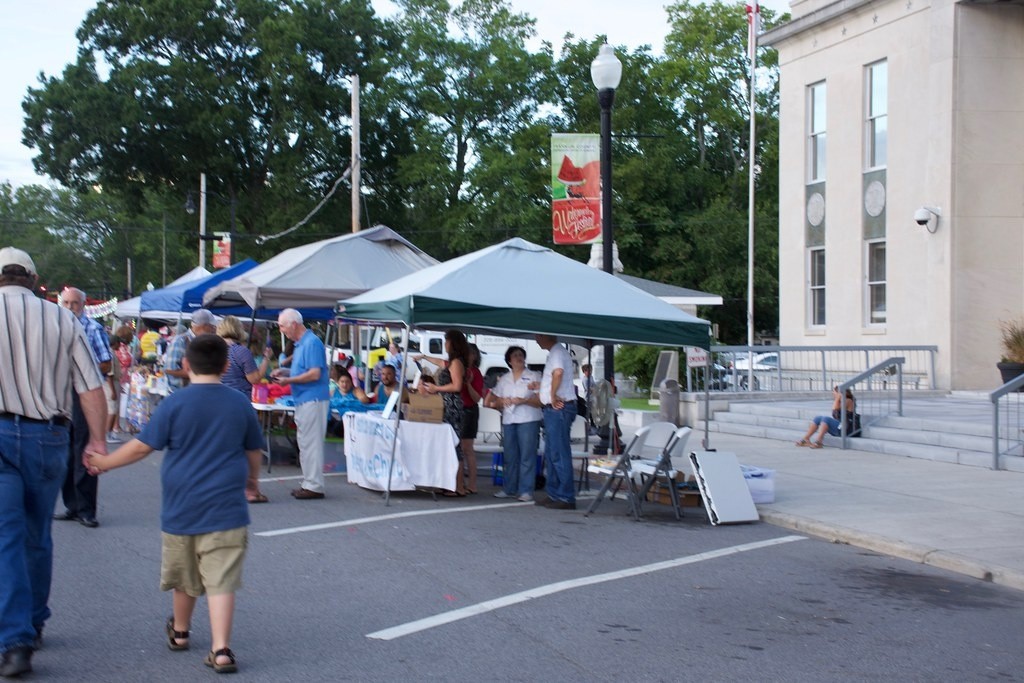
[997,320,1024,392]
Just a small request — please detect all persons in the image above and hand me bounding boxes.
[85,333,268,673]
[0,246,109,677]
[795,386,856,449]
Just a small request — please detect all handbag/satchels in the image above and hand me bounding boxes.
[838,410,861,437]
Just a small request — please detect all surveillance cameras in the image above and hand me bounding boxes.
[913,209,929,225]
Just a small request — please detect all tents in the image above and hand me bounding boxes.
[130,258,357,383]
[112,266,213,372]
[328,236,712,507]
[52,287,597,528]
[202,224,443,469]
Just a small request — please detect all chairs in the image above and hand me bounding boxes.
[585,422,693,522]
[472,407,590,492]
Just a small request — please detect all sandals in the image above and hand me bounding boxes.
[164,615,189,651]
[246,492,269,503]
[203,648,237,673]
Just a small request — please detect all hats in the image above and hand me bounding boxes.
[0,246,36,276]
[191,308,219,326]
[109,335,127,343]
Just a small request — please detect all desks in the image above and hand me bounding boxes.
[251,402,299,474]
[341,412,461,502]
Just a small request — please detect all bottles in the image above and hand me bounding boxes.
[257,388,267,403]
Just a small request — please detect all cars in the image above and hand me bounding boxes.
[711,351,781,395]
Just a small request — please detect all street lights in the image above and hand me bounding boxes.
[589,43,627,458]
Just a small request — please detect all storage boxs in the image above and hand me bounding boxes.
[400,388,444,424]
[490,448,545,487]
[740,464,776,504]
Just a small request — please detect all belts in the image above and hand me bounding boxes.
[0,411,70,428]
[543,401,574,408]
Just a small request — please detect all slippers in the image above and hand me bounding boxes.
[443,490,467,497]
[435,488,447,495]
[466,488,478,495]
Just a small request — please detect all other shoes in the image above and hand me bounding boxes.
[795,438,811,446]
[545,497,576,510]
[0,647,33,676]
[34,628,44,648]
[78,515,99,527]
[493,490,516,498]
[518,493,533,502]
[535,495,553,506]
[52,509,77,520]
[105,432,122,444]
[292,488,325,499]
[810,441,823,448]
